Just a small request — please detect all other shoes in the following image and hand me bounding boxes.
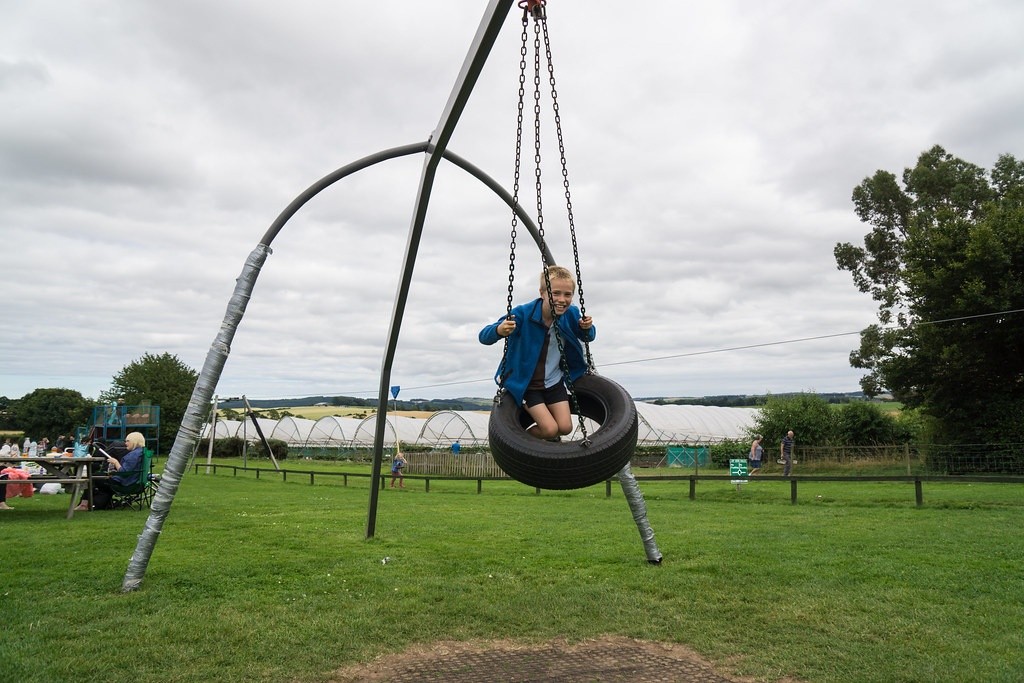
[0,506,14,510]
[73,504,88,511]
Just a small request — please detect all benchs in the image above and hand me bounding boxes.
[0,479,89,484]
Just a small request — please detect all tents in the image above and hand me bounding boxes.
[201,397,773,467]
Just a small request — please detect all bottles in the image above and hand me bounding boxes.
[11,444,19,457]
[23,438,30,457]
[52,446,57,453]
[29,442,37,457]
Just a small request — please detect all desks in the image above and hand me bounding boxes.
[0,457,106,520]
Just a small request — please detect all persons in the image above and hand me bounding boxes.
[748,435,765,476]
[450,440,461,455]
[0,433,105,510]
[391,453,408,488]
[72,432,147,511]
[780,431,796,480]
[479,266,597,440]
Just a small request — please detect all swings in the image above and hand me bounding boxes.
[489,3,638,490]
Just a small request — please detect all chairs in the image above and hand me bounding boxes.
[105,447,153,510]
